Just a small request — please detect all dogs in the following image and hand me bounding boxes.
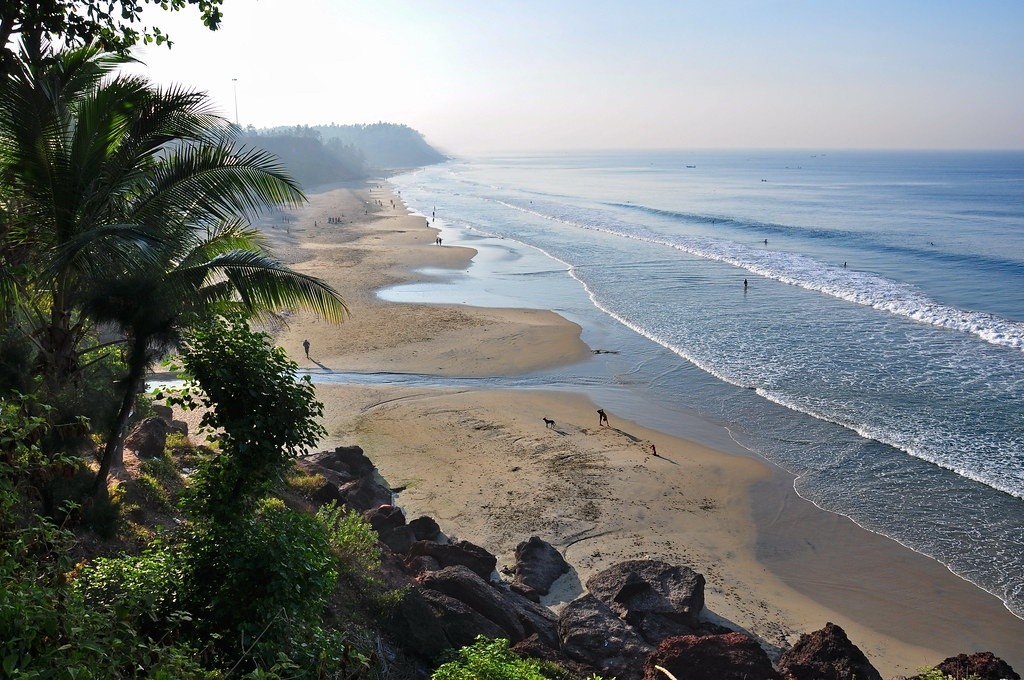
[543,417,556,429]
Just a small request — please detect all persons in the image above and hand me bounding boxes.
[303,340,310,358]
[281,177,442,246]
[597,409,609,426]
